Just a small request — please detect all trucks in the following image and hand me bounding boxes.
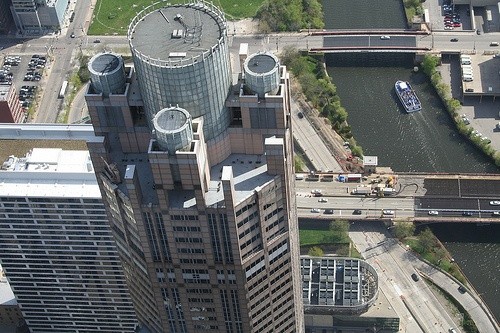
[491,41,499,46]
[351,185,372,195]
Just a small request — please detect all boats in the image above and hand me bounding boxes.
[394,80,423,113]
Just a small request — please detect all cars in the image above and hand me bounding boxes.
[72,31,74,38]
[461,113,489,144]
[318,198,328,202]
[450,38,458,42]
[94,39,100,43]
[311,209,321,213]
[381,36,391,40]
[492,212,500,216]
[299,112,303,118]
[354,210,361,214]
[0,52,47,106]
[461,53,473,81]
[428,210,439,215]
[324,209,333,214]
[489,200,500,205]
[311,189,322,194]
[463,212,473,216]
[383,210,394,215]
[442,1,462,29]
